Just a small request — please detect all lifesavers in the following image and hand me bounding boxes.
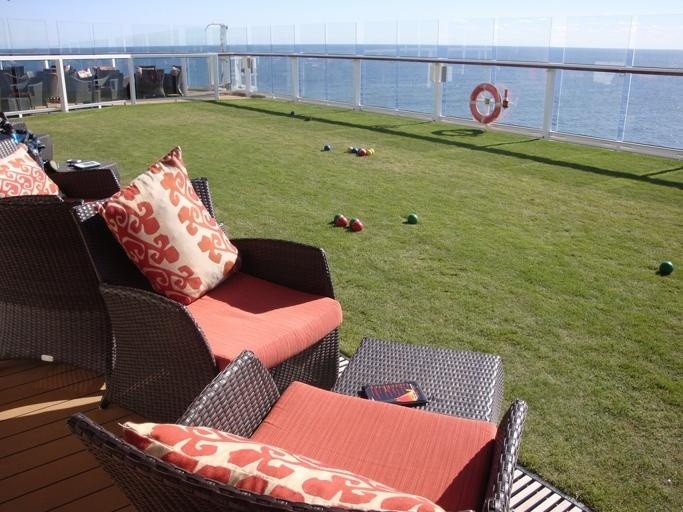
[470,82,501,123]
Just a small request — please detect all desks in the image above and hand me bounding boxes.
[50,158,120,200]
[0,139,121,375]
[52,158,120,184]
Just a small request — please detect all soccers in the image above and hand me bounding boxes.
[334,213,364,231]
[659,261,674,275]
[324,145,329,149]
[347,146,376,156]
[292,111,296,115]
[407,214,417,224]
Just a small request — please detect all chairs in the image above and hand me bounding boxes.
[0,119,54,162]
[0,65,185,113]
[66,335,529,512]
[71,175,339,422]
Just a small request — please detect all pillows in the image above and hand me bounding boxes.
[94,146,242,306]
[0,147,60,198]
[116,418,446,512]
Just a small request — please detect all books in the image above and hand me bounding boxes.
[360,381,427,406]
